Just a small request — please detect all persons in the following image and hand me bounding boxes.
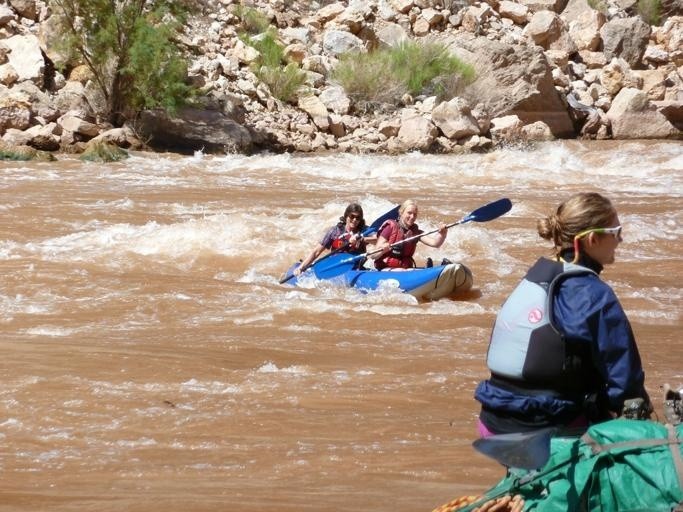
[472,189,654,470]
[370,198,452,274]
[293,203,377,277]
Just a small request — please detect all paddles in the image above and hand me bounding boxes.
[314,198,512,281]
[280,205,401,285]
[473,428,588,470]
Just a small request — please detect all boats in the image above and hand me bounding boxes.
[279,256,476,303]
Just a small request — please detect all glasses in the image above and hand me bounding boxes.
[570,225,621,264]
[346,212,363,221]
